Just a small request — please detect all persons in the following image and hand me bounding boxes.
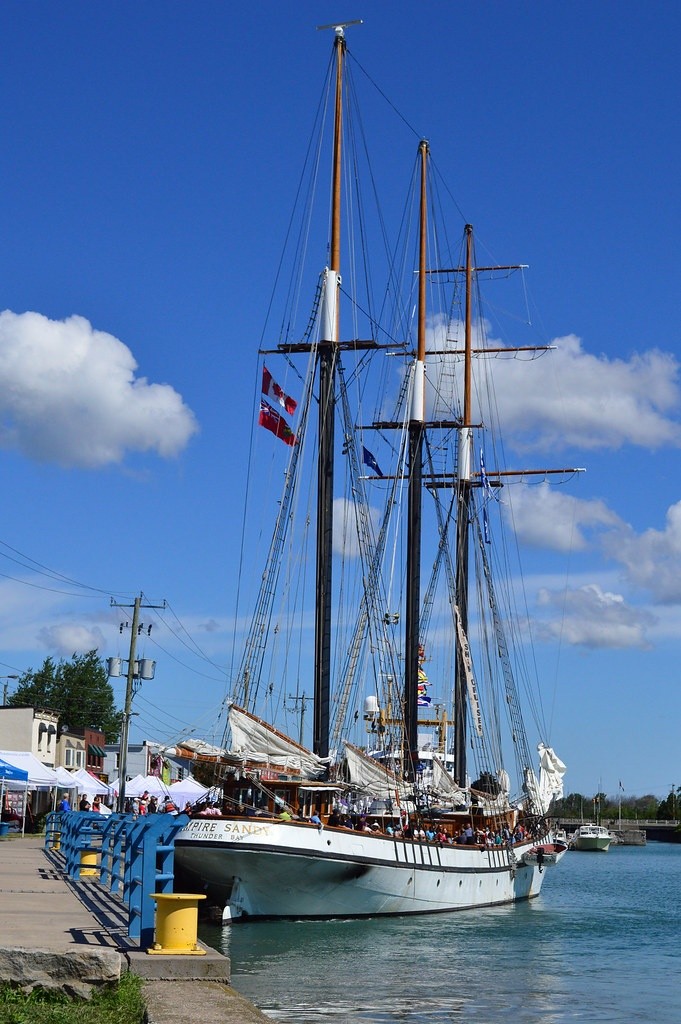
[59,793,71,812]
[255,805,547,846]
[79,794,91,811]
[132,791,244,816]
[92,797,101,811]
[395,760,426,782]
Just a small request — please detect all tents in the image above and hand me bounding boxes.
[109,778,142,797]
[52,765,97,811]
[128,775,209,812]
[72,768,117,812]
[0,750,58,837]
[0,759,28,839]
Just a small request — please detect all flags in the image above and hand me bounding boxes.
[262,366,297,416]
[417,644,433,706]
[362,446,383,477]
[259,397,296,446]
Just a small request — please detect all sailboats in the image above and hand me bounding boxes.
[122,19,577,916]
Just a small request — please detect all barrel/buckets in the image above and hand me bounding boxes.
[0,824,9,836]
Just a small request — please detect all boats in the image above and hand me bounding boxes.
[574,823,612,853]
